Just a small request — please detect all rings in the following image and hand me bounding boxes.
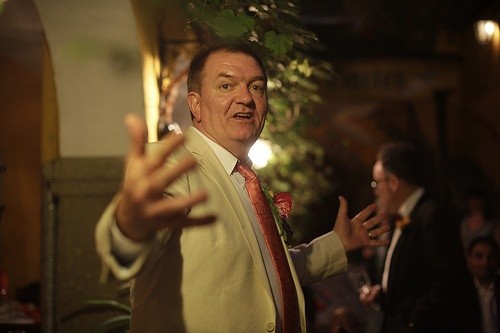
[368,231,373,237]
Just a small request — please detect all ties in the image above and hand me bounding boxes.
[234,163,302,333]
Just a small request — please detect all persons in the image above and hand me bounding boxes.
[468,235,500,333]
[359,142,481,333]
[96,41,390,333]
[456,180,500,260]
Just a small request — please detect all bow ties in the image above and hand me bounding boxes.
[394,216,411,229]
